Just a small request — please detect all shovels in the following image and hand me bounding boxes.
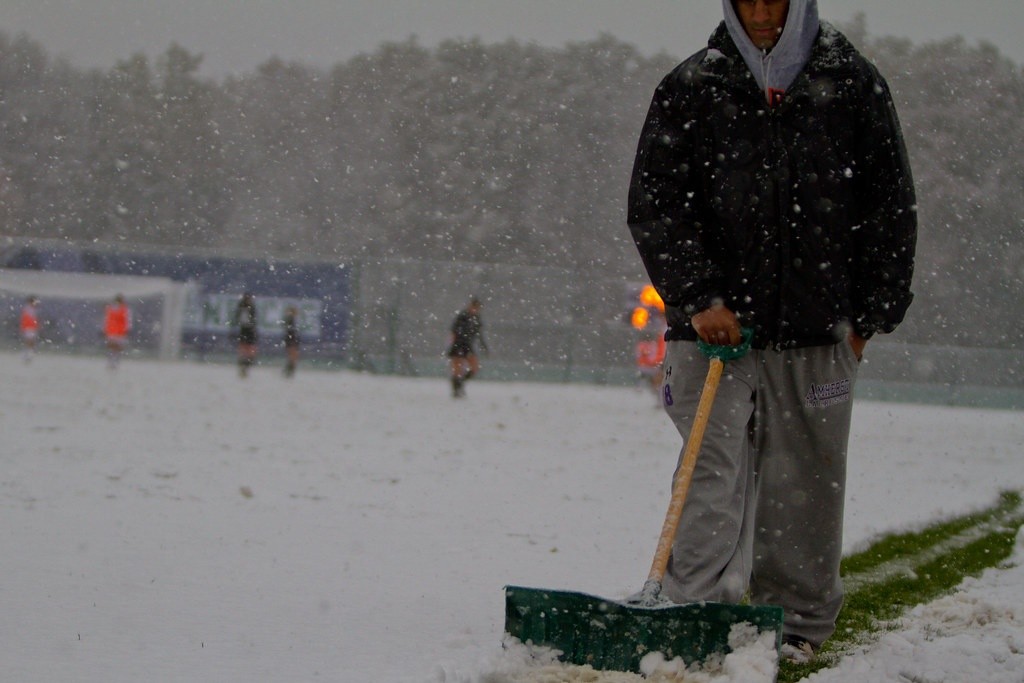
[504,328,787,683]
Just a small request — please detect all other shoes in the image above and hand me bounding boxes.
[782,633,814,657]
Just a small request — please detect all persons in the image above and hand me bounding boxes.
[281,306,302,374]
[625,0,920,646]
[229,292,258,363]
[18,296,41,347]
[447,296,484,399]
[105,293,130,353]
[635,332,667,409]
